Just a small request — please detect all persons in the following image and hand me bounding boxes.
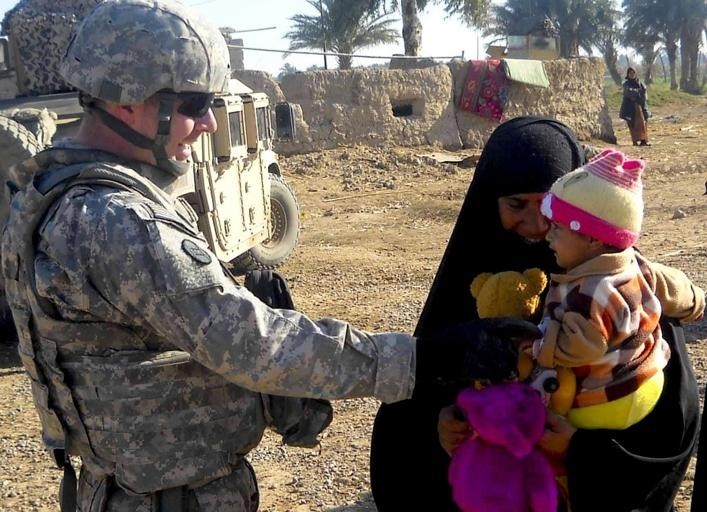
[618,67,652,147]
[0,0,546,512]
[532,147,706,431]
[368,113,700,512]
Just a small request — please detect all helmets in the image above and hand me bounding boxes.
[56,0,232,107]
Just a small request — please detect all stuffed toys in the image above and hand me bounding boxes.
[451,381,559,512]
[468,264,549,388]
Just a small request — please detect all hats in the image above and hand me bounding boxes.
[539,147,651,251]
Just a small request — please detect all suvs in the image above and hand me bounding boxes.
[0,0,300,270]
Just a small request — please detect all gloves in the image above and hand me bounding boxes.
[413,314,543,385]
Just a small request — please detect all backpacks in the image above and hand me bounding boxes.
[241,268,334,456]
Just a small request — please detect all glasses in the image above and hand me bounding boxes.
[159,90,216,117]
